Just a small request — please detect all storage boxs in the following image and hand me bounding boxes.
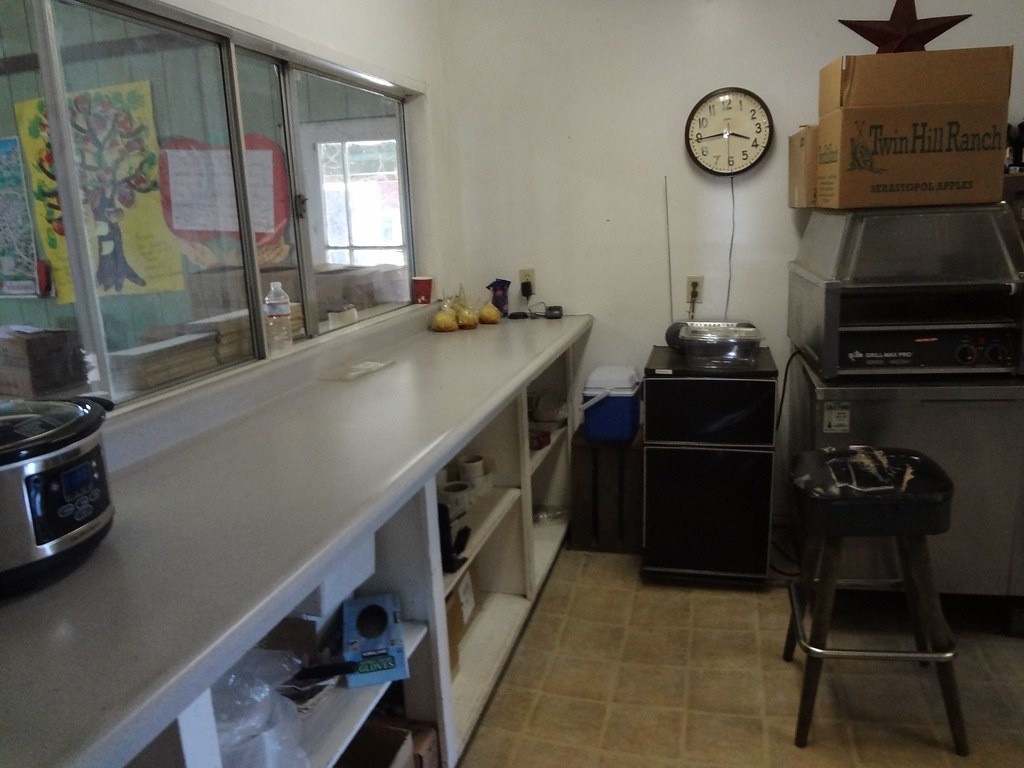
[789,125,819,211]
[444,556,483,684]
[286,528,410,688]
[816,45,1014,209]
[578,365,640,443]
[0,325,84,397]
[337,712,441,767]
[190,259,409,316]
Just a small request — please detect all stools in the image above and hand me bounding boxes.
[781,446,969,757]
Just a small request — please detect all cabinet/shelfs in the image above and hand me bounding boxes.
[0,314,594,768]
[640,346,779,586]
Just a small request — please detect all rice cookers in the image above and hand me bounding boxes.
[0,395,115,600]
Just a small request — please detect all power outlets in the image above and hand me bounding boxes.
[686,275,704,303]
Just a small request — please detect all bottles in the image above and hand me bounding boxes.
[533,505,573,525]
[265,282,293,355]
[1004,120,1024,172]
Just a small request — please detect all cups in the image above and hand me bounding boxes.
[439,479,475,514]
[411,276,433,306]
[458,455,490,511]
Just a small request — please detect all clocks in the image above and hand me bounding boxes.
[685,86,774,176]
